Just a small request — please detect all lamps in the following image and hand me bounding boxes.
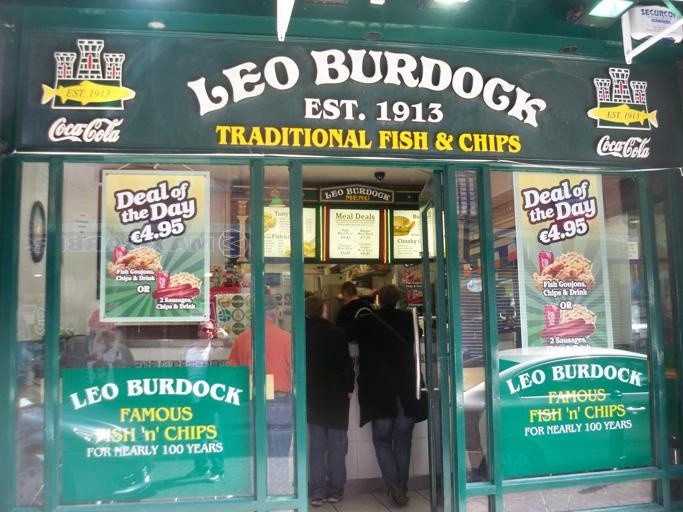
[565,0,640,31]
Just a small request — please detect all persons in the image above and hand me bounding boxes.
[59,310,136,376]
[226,297,293,497]
[356,284,422,507]
[336,279,374,344]
[305,290,355,507]
[170,319,234,483]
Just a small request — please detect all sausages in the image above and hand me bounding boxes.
[153,283,200,304]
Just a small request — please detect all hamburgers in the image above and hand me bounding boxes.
[394,216,411,236]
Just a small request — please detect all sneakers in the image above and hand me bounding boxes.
[310,496,342,507]
[387,486,411,506]
